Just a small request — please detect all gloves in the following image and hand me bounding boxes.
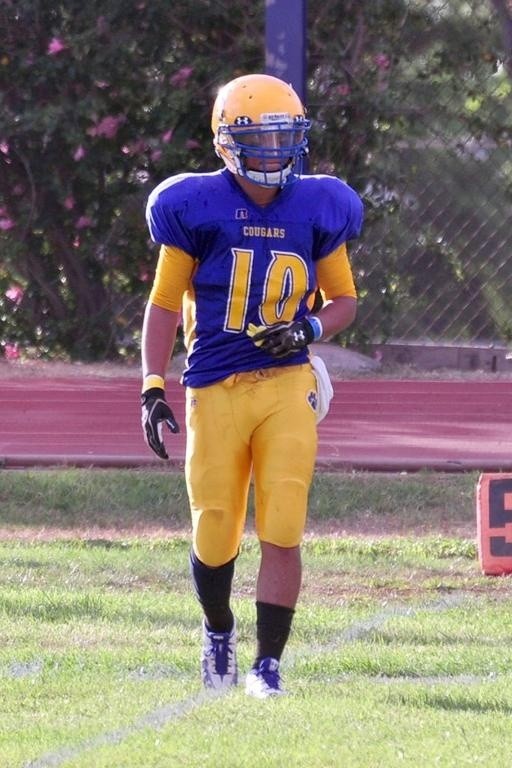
[252,316,316,360]
[140,387,180,459]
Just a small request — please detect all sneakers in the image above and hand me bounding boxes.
[245,657,288,699]
[201,612,238,694]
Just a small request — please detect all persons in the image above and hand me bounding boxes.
[139,70,368,706]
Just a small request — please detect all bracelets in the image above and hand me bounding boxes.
[308,316,323,342]
[141,374,165,394]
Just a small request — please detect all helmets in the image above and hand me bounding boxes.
[212,74,311,188]
